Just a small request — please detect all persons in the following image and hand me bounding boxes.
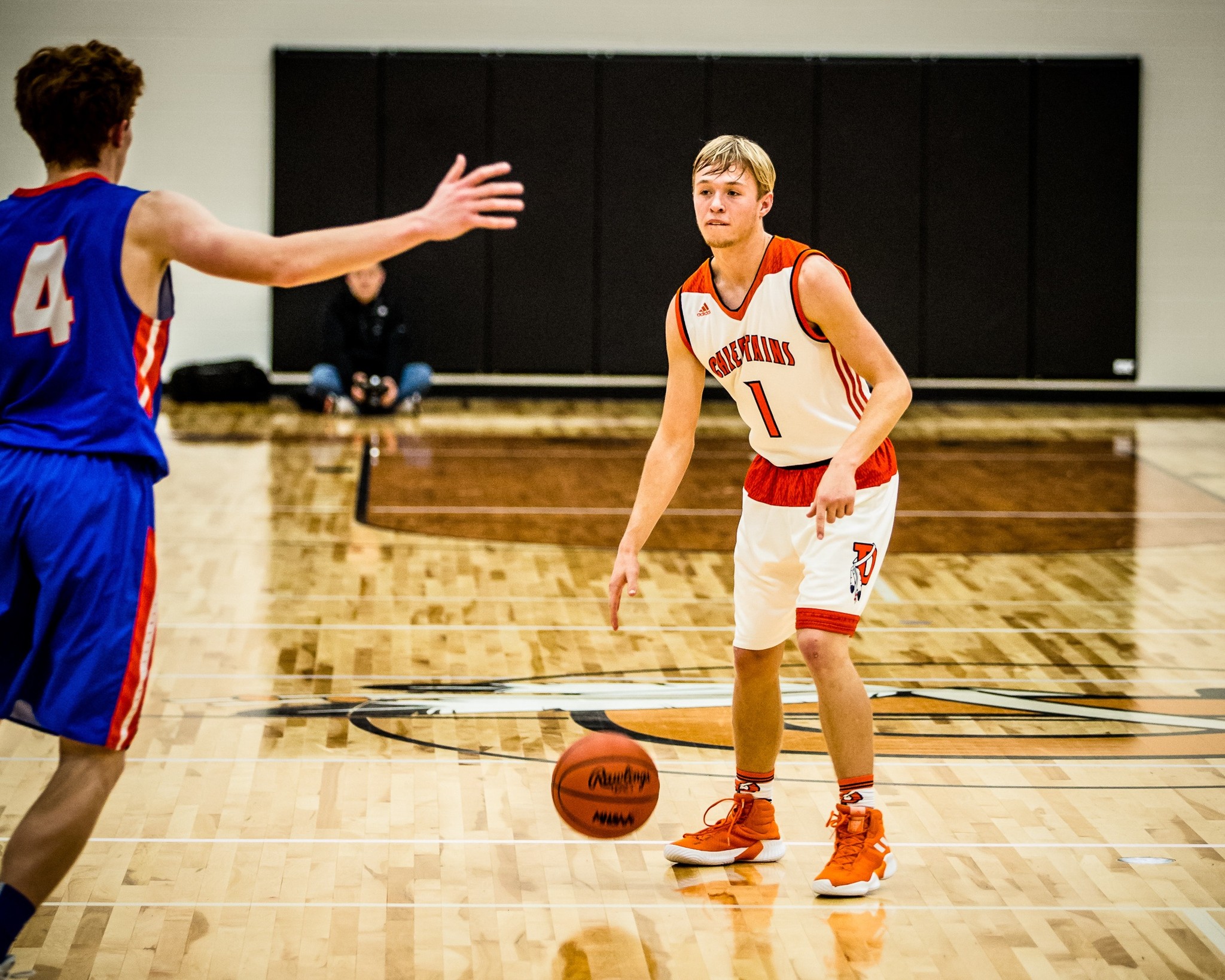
[0,39,525,979]
[608,135,914,898]
[308,264,430,418]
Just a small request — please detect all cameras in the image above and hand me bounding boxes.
[360,383,389,406]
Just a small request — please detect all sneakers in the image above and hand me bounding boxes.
[812,803,897,896]
[664,793,786,865]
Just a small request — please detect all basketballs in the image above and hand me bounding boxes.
[551,731,661,839]
